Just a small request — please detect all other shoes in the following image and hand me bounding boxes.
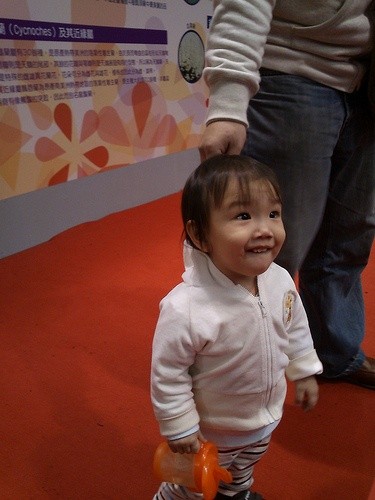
[239,488,263,498]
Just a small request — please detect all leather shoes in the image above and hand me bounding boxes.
[337,357,374,389]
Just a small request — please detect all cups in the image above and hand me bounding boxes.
[154,440,232,500]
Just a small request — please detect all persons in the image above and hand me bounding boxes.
[150,154,324,499]
[198,0,375,390]
